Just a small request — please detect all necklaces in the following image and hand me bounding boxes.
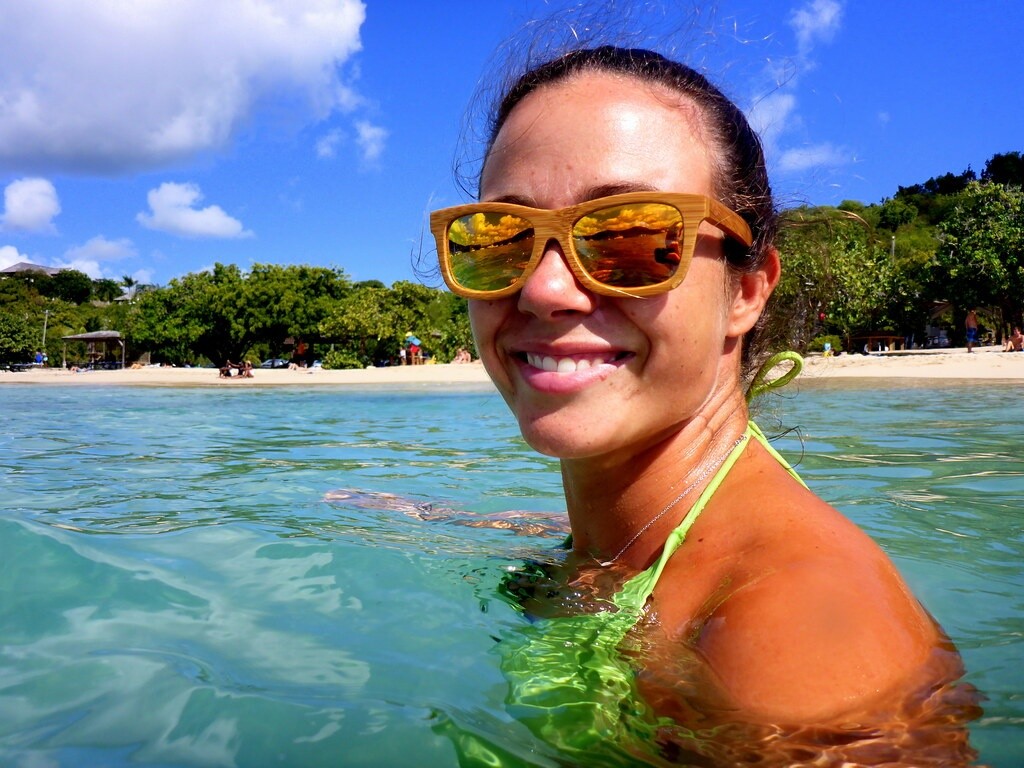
[587,429,746,568]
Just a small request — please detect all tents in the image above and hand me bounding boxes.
[60,331,126,372]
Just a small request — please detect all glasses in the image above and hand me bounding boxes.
[430,191,753,297]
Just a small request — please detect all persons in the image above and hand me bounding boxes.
[1006,327,1024,353]
[450,347,470,365]
[399,344,407,365]
[219,359,253,378]
[71,366,90,375]
[964,309,977,353]
[131,362,152,370]
[429,45,930,718]
[297,342,305,359]
[289,360,308,370]
